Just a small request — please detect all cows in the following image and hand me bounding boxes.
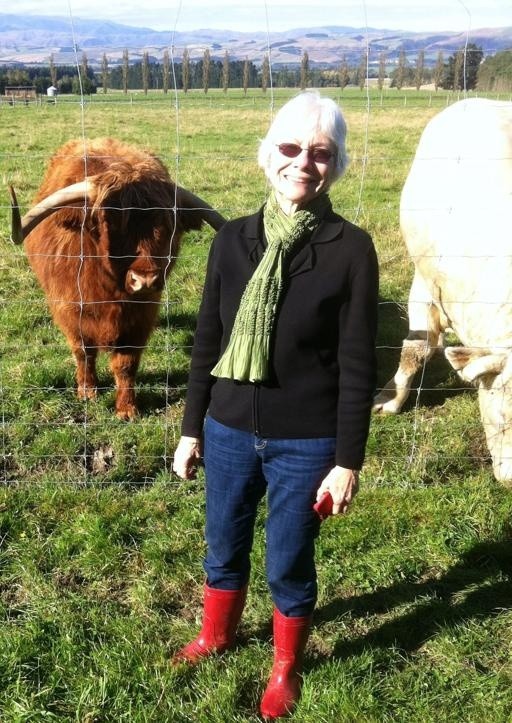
[8,135,227,423]
[370,97,512,491]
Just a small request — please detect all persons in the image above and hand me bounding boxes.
[172,89,380,720]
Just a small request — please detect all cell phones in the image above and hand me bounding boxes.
[312,492,333,521]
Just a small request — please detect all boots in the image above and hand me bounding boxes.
[176,573,249,665]
[259,603,312,721]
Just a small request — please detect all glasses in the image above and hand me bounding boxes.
[274,142,337,163]
